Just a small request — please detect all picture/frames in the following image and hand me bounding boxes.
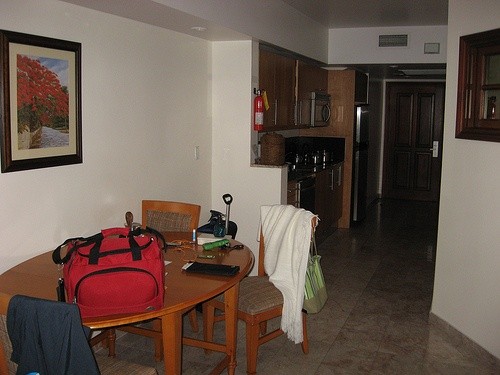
[0,29,82,172]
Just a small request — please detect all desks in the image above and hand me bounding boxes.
[0,232,255,375]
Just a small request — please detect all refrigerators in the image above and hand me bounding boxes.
[351,105,366,222]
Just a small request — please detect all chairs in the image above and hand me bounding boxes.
[0,293,157,375]
[202,205,316,375]
[142,200,201,361]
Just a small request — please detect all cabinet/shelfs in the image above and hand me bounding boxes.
[257,42,368,130]
[289,162,342,236]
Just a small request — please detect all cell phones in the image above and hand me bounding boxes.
[186,262,240,275]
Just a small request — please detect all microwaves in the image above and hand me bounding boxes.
[311,92,331,127]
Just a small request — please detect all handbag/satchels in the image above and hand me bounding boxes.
[63,236,164,322]
[303,228,328,313]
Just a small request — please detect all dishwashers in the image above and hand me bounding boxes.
[295,174,315,215]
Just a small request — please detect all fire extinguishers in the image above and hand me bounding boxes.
[254,88,265,130]
